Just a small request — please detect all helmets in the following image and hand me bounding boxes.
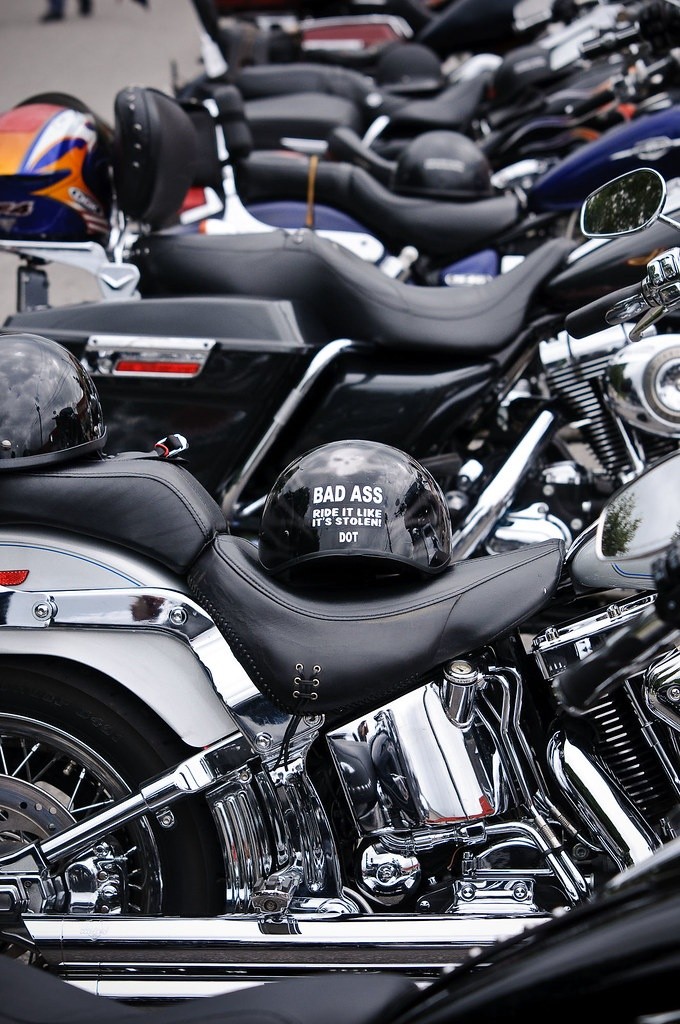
[256,437,454,573]
[374,43,446,92]
[0,331,109,469]
[387,129,496,199]
[0,90,114,241]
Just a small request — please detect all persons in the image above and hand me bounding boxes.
[41,0,93,22]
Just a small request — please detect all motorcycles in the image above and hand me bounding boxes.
[0,0,680,1024]
